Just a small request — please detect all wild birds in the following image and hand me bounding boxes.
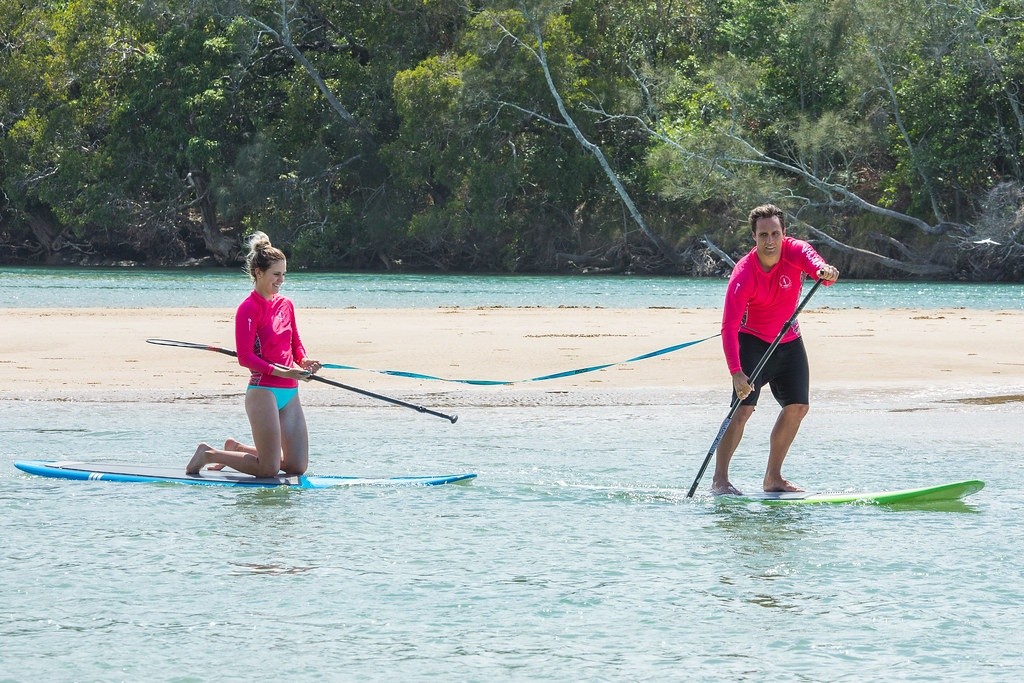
[394,258,402,266]
[972,238,1003,247]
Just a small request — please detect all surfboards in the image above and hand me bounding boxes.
[569,478,985,505]
[15,459,478,488]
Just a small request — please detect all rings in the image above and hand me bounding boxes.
[739,391,743,395]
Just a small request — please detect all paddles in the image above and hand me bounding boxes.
[683,269,825,499]
[146,338,458,424]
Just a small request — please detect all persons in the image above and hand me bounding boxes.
[708,204,839,494]
[184,232,322,478]
